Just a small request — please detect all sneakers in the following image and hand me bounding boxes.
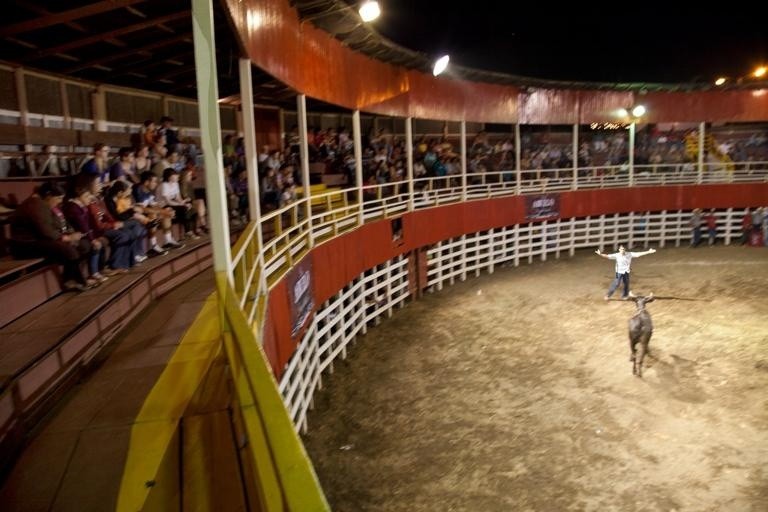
[50,227,208,292]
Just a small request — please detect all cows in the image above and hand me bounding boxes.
[626,290,656,378]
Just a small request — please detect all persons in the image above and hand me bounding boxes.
[11,116,768,291]
[595,243,656,300]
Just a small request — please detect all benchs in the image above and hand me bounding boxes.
[296,161,349,207]
[1,175,288,511]
